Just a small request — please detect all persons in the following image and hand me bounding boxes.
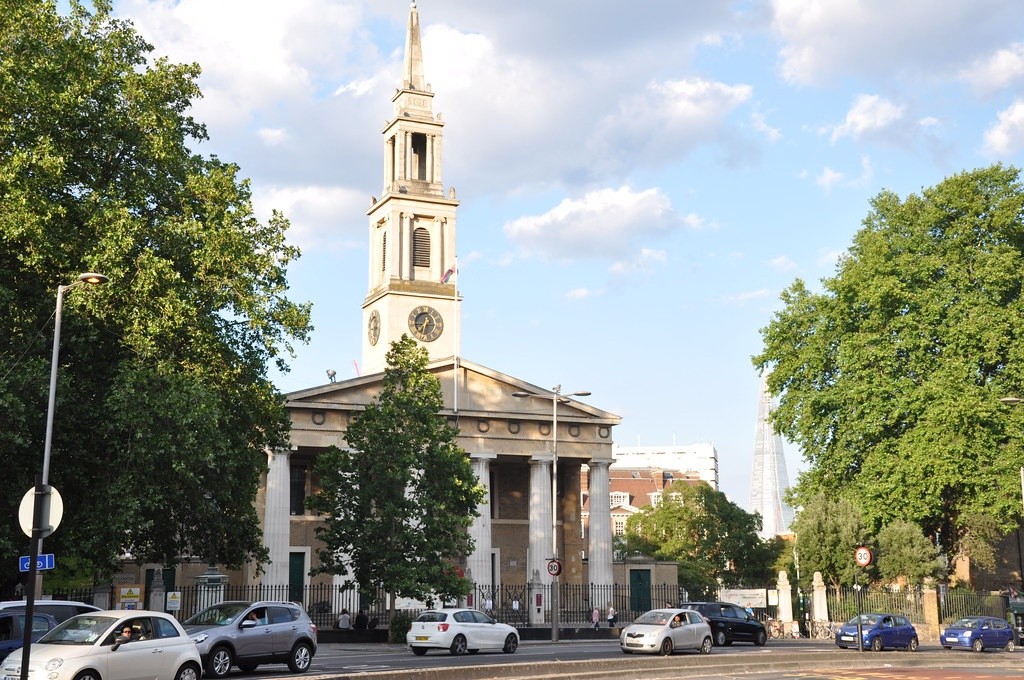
[352,609,368,629]
[607,601,615,627]
[246,611,262,625]
[338,608,351,629]
[674,614,686,626]
[592,606,600,627]
[121,625,135,641]
[982,623,988,629]
[744,602,754,617]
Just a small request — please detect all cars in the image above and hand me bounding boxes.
[620,608,713,656]
[406,608,520,656]
[835,613,919,652]
[941,616,1016,653]
[0,611,70,666]
[1017,622,1024,646]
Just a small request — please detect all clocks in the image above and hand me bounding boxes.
[368,309,380,346]
[408,306,443,342]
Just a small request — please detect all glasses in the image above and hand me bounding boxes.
[123,631,131,634]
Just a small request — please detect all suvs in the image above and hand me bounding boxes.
[181,600,318,678]
[0,609,203,680]
[679,601,768,647]
[0,599,105,624]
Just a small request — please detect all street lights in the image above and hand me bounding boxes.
[513,383,591,645]
[20,272,110,680]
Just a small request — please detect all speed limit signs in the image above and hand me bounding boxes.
[855,547,871,567]
[547,561,561,576]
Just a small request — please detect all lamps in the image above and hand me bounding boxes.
[326,370,336,383]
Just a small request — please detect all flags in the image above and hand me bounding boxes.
[440,264,455,285]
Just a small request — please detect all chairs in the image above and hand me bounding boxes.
[131,620,152,642]
[463,613,471,619]
